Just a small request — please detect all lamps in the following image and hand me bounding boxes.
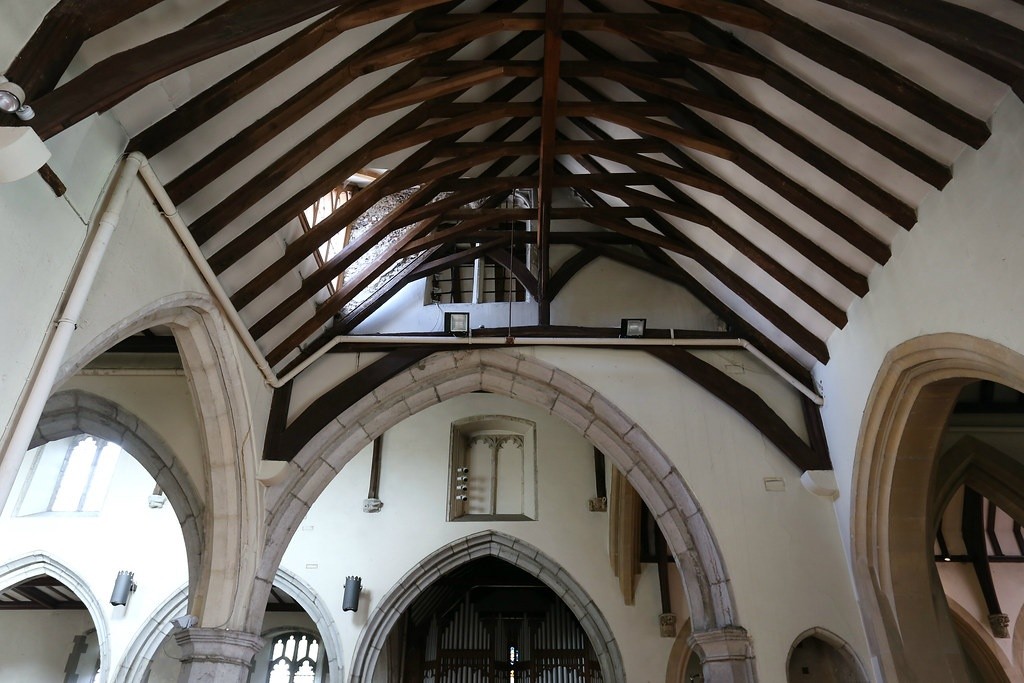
[444,311,470,337]
[343,575,362,612]
[109,571,137,606]
[0,83,35,121]
[619,319,647,337]
